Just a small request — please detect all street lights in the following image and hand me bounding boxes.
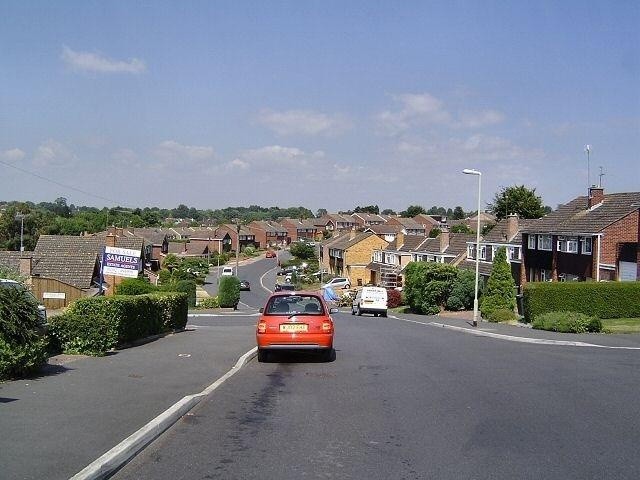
[463,169,482,327]
[14,211,24,257]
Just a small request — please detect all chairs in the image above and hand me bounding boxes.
[275,302,290,310]
[304,302,319,313]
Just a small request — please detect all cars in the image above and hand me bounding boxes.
[274,262,351,293]
[239,279,251,291]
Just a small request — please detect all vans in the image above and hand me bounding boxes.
[222,266,234,278]
[350,287,388,318]
[0,279,48,329]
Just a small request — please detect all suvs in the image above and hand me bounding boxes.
[266,251,277,258]
[306,242,315,247]
[255,291,339,361]
